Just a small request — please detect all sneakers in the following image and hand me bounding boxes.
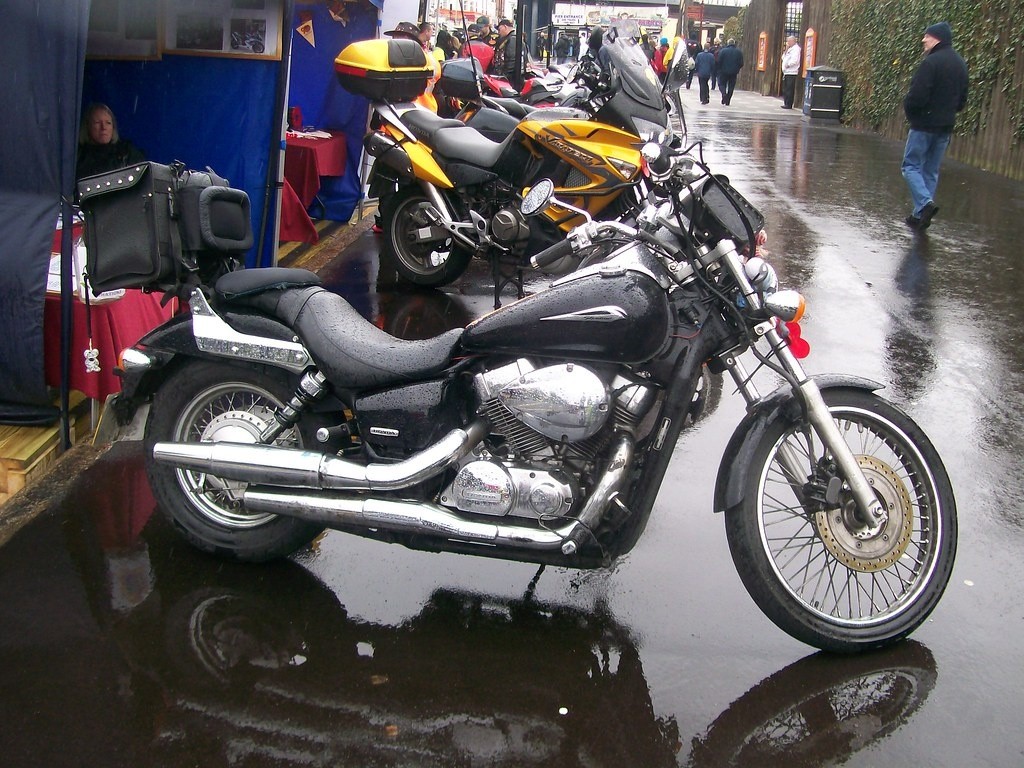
[918,201,938,230]
[906,215,919,225]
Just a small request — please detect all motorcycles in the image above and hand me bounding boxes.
[332,22,691,311]
[72,34,962,652]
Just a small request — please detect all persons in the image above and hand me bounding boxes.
[694,43,715,105]
[709,37,723,90]
[900,23,969,230]
[717,38,744,106]
[573,35,581,62]
[385,15,529,119]
[781,36,801,109]
[706,36,711,43]
[536,32,545,61]
[686,31,701,89]
[75,102,142,206]
[554,31,571,65]
[639,34,679,115]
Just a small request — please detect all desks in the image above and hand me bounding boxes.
[279,127,348,247]
[44,220,191,436]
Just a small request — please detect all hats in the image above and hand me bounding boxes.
[468,24,479,33]
[477,16,489,27]
[383,21,425,48]
[660,38,667,44]
[495,19,511,30]
[925,21,951,42]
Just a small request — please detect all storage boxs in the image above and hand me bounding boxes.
[77,162,230,298]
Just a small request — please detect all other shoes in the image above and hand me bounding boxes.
[726,101,729,105]
[781,104,792,109]
[702,100,709,104]
[722,94,727,104]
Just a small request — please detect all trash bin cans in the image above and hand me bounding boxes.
[800,65,842,125]
